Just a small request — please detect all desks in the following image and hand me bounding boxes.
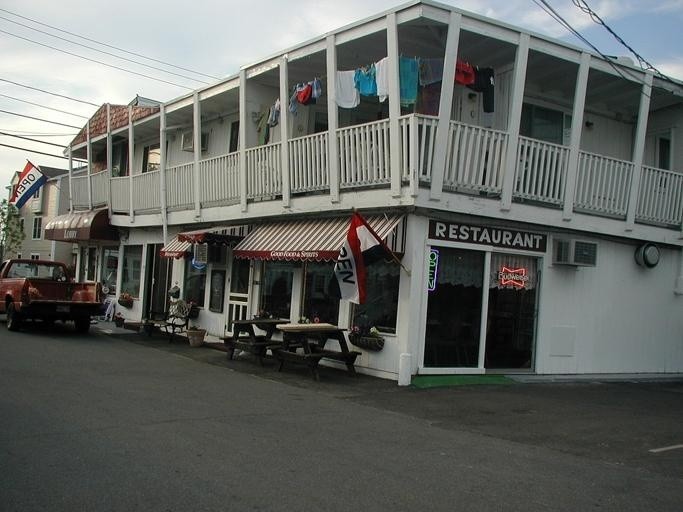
[226,319,290,362]
[275,323,347,382]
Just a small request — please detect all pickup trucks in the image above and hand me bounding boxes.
[0,259,104,333]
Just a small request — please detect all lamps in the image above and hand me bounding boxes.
[634,242,658,268]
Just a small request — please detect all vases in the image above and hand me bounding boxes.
[348,334,384,352]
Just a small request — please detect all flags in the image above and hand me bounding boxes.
[325,211,381,306]
[7,161,46,211]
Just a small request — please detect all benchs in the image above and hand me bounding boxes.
[219,335,362,381]
[147,299,192,346]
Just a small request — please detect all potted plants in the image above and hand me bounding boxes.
[117,291,132,308]
[114,312,123,327]
[185,325,206,347]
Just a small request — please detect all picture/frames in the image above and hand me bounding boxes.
[209,269,226,313]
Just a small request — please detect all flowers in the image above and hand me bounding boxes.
[351,324,382,336]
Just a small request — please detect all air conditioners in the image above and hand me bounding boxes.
[181,132,207,151]
[552,239,598,267]
